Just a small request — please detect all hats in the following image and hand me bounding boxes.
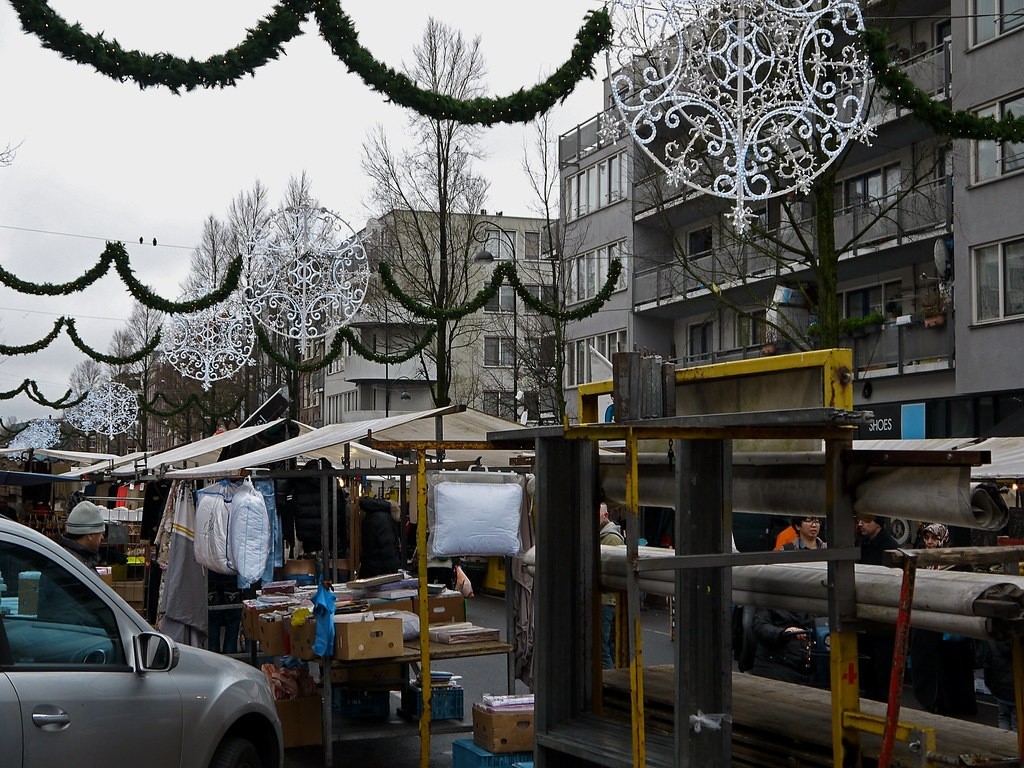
[66,500,105,534]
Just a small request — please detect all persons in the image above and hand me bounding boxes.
[908,523,981,723]
[37,500,106,627]
[751,606,822,687]
[0,496,16,522]
[854,516,902,568]
[984,622,1018,734]
[358,497,401,579]
[425,528,461,591]
[773,516,827,551]
[600,504,625,668]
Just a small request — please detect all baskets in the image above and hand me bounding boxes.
[452,739,534,768]
[800,648,871,690]
[403,686,464,720]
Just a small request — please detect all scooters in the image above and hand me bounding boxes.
[773,617,834,690]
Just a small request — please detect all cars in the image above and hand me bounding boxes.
[0,515,285,768]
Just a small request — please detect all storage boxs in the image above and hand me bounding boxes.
[240,607,287,640]
[472,702,536,753]
[402,684,464,719]
[332,666,348,691]
[258,617,284,655]
[287,620,317,659]
[348,667,369,688]
[371,663,402,689]
[333,617,405,661]
[452,737,533,768]
[275,695,324,748]
[369,599,413,613]
[413,595,464,623]
[773,284,809,304]
[112,579,145,601]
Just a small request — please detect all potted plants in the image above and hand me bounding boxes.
[806,308,887,343]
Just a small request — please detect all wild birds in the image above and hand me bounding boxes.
[139,236,144,245]
[153,237,158,246]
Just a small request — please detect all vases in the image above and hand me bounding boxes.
[111,563,128,581]
[762,341,779,353]
[128,563,144,581]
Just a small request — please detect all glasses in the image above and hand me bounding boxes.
[802,520,822,526]
[924,534,937,540]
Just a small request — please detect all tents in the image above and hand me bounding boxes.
[855,437,1024,478]
[0,403,615,481]
